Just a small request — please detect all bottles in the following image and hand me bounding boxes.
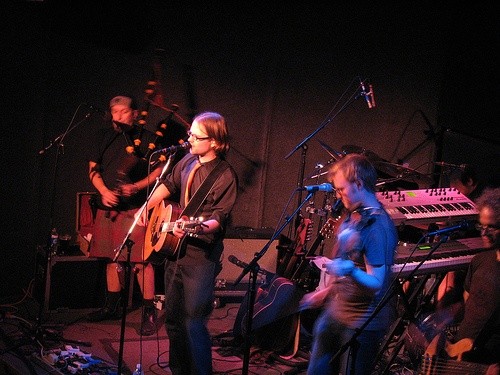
[133,364,144,375]
[51,228,58,238]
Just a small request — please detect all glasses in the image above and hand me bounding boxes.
[187,130,212,141]
[474,223,500,233]
[332,182,355,195]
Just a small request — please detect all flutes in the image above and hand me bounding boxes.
[88,80,181,211]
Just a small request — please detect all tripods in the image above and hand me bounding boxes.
[1,117,94,353]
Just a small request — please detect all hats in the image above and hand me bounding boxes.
[109,96,137,110]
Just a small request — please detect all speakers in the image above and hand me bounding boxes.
[212,237,279,286]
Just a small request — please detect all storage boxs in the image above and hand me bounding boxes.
[44,192,108,313]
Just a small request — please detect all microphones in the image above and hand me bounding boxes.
[156,141,192,152]
[360,81,376,107]
[300,182,335,192]
[434,160,469,171]
[424,222,469,238]
[227,254,250,267]
[88,102,112,121]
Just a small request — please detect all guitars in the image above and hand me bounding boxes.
[141,198,204,262]
[232,217,338,340]
[417,332,500,375]
[253,218,314,304]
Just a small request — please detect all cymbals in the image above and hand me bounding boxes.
[370,161,434,181]
[316,137,342,163]
[339,143,385,162]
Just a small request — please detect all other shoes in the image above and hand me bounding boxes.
[141,313,157,336]
[85,305,126,321]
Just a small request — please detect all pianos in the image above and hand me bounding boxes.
[389,236,484,278]
[375,186,480,227]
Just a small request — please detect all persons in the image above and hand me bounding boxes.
[134,112,238,375]
[437,168,485,302]
[303,153,398,375]
[87,95,167,334]
[455,189,500,363]
